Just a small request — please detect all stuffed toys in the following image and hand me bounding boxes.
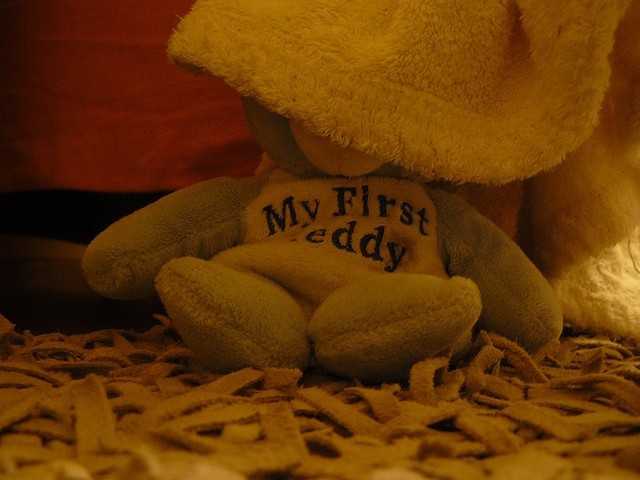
[80,92,563,381]
